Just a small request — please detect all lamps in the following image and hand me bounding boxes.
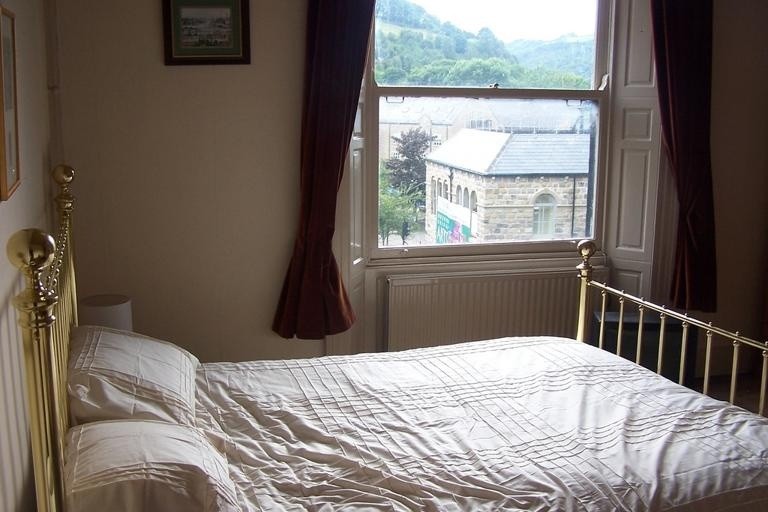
[76,294,134,333]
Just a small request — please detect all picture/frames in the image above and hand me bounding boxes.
[0,5,23,201]
[161,0,251,66]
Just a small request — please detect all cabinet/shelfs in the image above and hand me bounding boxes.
[592,310,699,390]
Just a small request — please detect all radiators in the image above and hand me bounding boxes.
[383,266,610,353]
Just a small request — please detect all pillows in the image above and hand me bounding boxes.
[65,325,200,426]
[63,419,243,512]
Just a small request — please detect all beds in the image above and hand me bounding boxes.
[6,165,768,512]
